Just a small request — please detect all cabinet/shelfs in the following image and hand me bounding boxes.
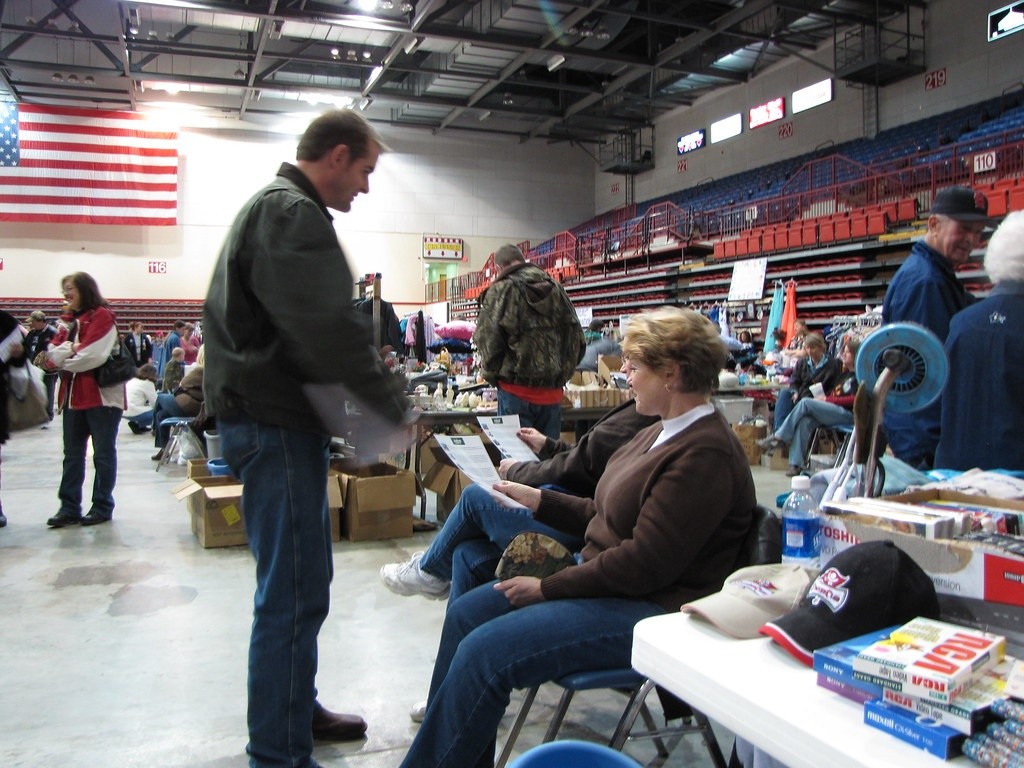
[885,223,993,299]
[678,240,886,328]
[451,301,481,322]
[559,270,678,331]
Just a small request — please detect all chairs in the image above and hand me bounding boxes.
[532,87,1024,269]
[0,299,205,338]
[487,501,784,768]
[155,400,206,473]
[505,738,644,768]
[806,425,854,468]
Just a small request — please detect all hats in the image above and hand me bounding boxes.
[25,310,46,323]
[930,186,999,227]
[758,538,941,669]
[680,562,820,639]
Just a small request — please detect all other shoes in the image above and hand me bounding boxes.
[755,434,786,450]
[128,420,142,434]
[311,707,367,742]
[151,449,169,460]
[151,429,156,437]
[0,514,7,528]
[785,458,808,477]
[141,426,151,432]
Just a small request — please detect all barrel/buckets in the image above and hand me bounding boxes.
[206,456,234,476]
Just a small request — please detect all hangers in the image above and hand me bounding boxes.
[404,307,422,317]
[832,314,882,327]
[361,291,372,299]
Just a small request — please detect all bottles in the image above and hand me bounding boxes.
[782,476,823,566]
[739,351,784,385]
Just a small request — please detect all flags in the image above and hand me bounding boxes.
[0,101,178,226]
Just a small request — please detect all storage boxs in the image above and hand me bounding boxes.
[815,672,877,705]
[760,454,773,468]
[732,422,767,465]
[328,455,425,541]
[421,439,503,524]
[808,453,836,476]
[821,487,1024,607]
[819,438,835,454]
[770,457,788,470]
[812,622,904,702]
[852,615,1007,705]
[862,697,1004,763]
[597,356,622,384]
[169,475,344,551]
[712,394,755,426]
[186,459,211,479]
[774,448,784,458]
[882,654,1017,738]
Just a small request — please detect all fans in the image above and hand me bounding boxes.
[852,320,951,498]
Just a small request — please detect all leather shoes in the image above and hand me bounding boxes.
[80,509,107,525]
[47,513,82,526]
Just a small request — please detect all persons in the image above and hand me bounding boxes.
[401,306,755,768]
[24,310,57,420]
[756,340,861,476]
[151,343,205,461]
[162,347,186,392]
[179,322,200,365]
[203,110,412,768]
[471,244,586,441]
[773,332,836,449]
[47,271,128,528]
[574,319,622,373]
[934,209,1024,472]
[381,352,661,722]
[880,186,987,471]
[740,330,754,356]
[125,322,153,368]
[0,309,24,527]
[158,320,186,378]
[126,366,158,434]
[786,320,815,349]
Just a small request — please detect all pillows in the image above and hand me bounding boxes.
[434,320,477,341]
[426,337,474,354]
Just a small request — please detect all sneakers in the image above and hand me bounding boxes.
[411,703,427,722]
[380,548,451,602]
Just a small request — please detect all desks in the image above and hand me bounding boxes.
[403,407,614,533]
[605,608,985,768]
[717,383,790,435]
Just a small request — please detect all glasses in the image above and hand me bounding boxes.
[61,285,75,293]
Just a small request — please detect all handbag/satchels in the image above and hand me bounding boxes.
[90,306,136,388]
[494,530,579,584]
[6,378,50,432]
